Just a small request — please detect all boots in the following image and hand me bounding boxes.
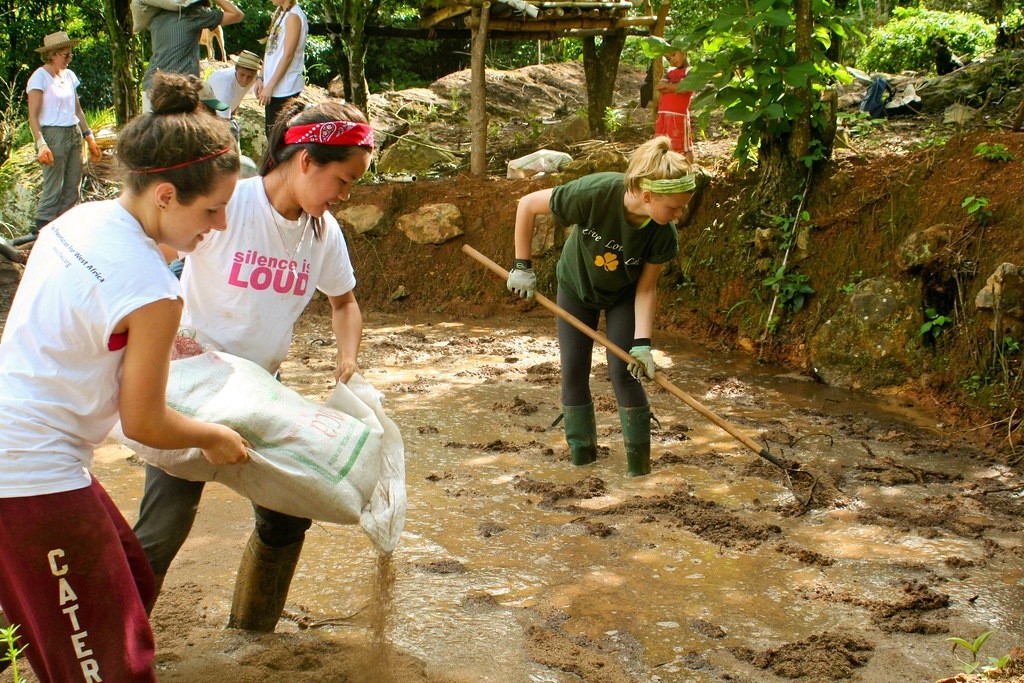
[618,403,661,477]
[562,402,597,467]
[227,528,305,633]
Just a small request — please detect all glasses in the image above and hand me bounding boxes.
[54,51,74,59]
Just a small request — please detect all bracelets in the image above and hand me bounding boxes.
[83,130,92,137]
[256,76,263,80]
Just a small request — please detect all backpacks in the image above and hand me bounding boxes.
[860,77,897,118]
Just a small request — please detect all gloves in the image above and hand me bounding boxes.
[626,338,654,383]
[37,138,54,166]
[85,134,102,164]
[507,259,536,301]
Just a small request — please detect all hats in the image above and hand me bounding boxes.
[34,32,80,53]
[229,50,263,70]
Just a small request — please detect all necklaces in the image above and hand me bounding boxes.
[263,180,309,262]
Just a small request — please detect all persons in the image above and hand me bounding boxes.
[507,135,696,478]
[196,80,239,142]
[208,50,262,118]
[140,0,245,115]
[133,85,373,633]
[0,72,251,683]
[654,39,693,163]
[25,31,101,235]
[254,0,309,142]
